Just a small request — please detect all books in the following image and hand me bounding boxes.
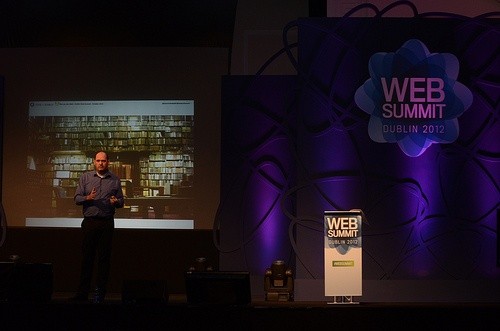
[30,115,194,213]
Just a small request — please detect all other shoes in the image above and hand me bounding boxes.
[67,294,88,303]
[95,294,105,304]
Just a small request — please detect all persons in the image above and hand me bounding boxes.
[72,151,123,304]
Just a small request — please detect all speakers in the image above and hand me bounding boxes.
[185,270,251,303]
[0,262,54,303]
